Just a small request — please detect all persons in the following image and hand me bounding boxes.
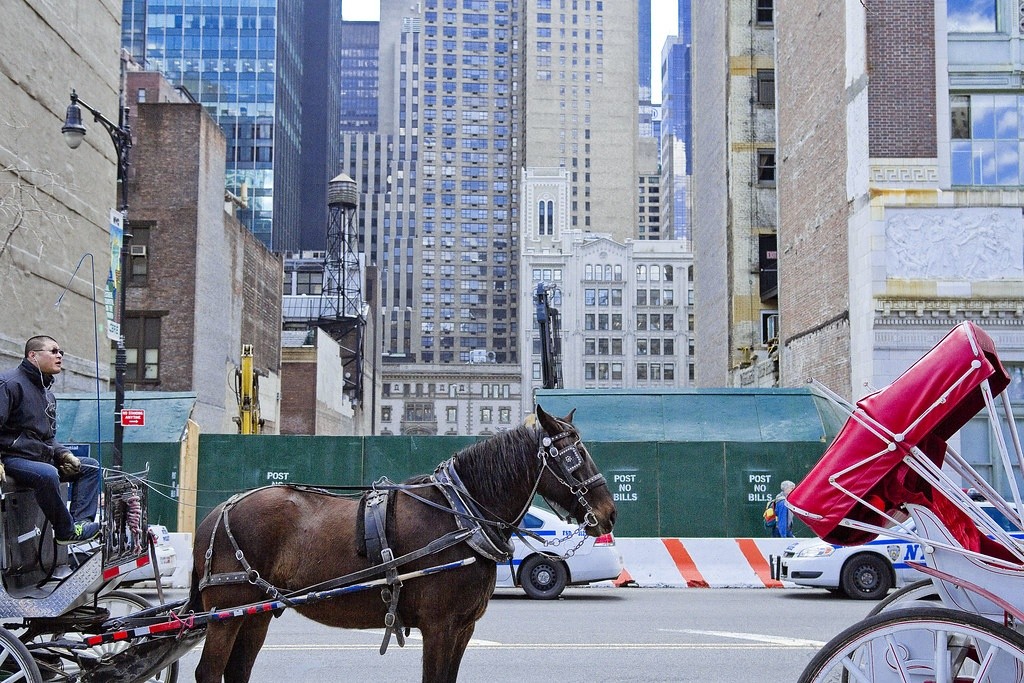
[772,481,795,538]
[0,336,100,545]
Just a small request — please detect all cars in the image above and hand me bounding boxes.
[64,498,179,590]
[768,484,1024,601]
[492,504,623,600]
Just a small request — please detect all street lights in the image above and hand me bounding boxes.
[58,87,134,477]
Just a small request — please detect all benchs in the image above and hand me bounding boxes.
[907,489,1024,615]
[0,475,68,576]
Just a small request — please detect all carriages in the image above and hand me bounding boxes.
[0,401,620,683]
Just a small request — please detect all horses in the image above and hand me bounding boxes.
[185,405,617,683]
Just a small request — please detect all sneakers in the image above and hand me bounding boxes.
[56,519,100,545]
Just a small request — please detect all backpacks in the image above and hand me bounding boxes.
[763,494,786,531]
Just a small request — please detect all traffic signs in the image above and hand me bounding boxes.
[120,408,146,428]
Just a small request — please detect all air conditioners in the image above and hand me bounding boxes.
[130,245,147,257]
[767,315,779,342]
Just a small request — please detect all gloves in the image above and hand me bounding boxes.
[0,464,6,483]
[60,453,82,477]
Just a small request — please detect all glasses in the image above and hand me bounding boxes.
[33,348,64,356]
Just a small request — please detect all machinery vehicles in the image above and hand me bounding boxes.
[227,343,266,434]
[531,280,564,389]
